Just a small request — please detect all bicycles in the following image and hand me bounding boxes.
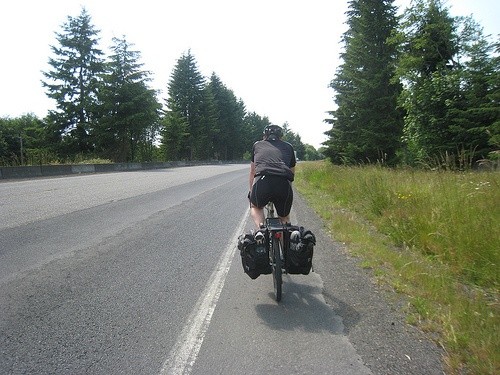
[263,202,288,302]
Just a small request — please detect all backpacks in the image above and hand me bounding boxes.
[283,226,316,275]
[238,228,273,279]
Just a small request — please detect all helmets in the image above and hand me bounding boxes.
[262,125,283,137]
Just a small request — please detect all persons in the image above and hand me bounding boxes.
[248,125,297,230]
